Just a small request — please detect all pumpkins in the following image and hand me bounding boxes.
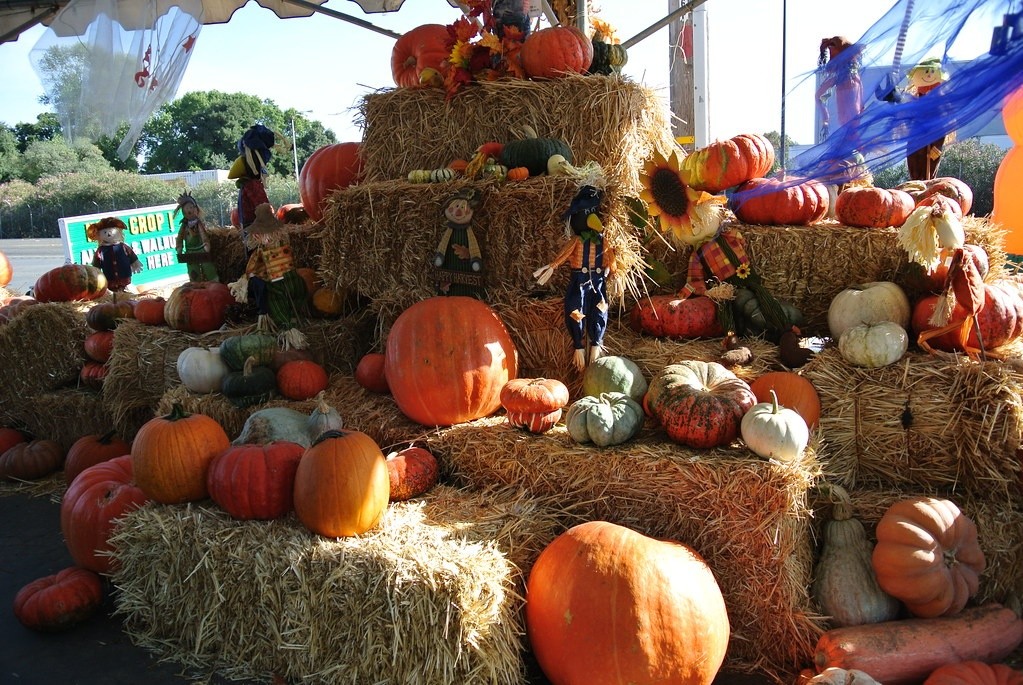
[0,133,1023,685]
[390,24,456,88]
[520,25,629,80]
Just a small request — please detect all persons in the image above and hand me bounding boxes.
[87,218,143,292]
[173,192,219,283]
[876,56,956,182]
[433,185,486,301]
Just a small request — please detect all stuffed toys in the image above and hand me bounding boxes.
[229,204,314,331]
[534,184,623,371]
[897,192,990,328]
[813,36,885,179]
[228,125,275,225]
[672,197,801,340]
